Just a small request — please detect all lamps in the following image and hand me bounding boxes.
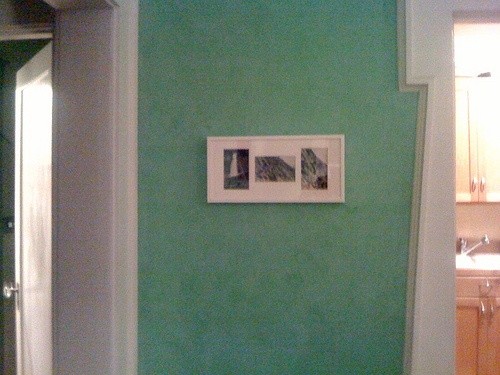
[456,72,496,200]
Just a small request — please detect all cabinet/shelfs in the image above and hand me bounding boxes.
[455,269,500,375]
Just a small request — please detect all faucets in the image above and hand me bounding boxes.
[459,235,490,256]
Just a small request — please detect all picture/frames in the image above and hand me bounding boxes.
[206,133,346,206]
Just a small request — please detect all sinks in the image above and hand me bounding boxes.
[456,253,500,270]
